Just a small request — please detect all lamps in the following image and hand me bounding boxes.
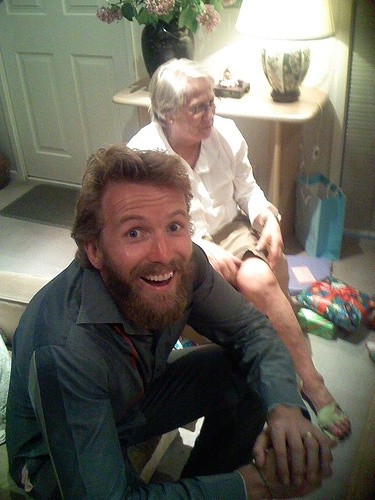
[234,0,337,104]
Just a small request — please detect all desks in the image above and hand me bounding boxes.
[107,77,329,242]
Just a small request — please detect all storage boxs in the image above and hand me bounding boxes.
[296,276,375,339]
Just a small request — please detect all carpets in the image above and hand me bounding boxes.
[0,184,81,231]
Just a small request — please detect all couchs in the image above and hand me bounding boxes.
[0,270,198,500]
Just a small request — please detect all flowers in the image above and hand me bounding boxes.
[95,0,237,33]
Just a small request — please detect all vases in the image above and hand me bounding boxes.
[141,21,195,79]
